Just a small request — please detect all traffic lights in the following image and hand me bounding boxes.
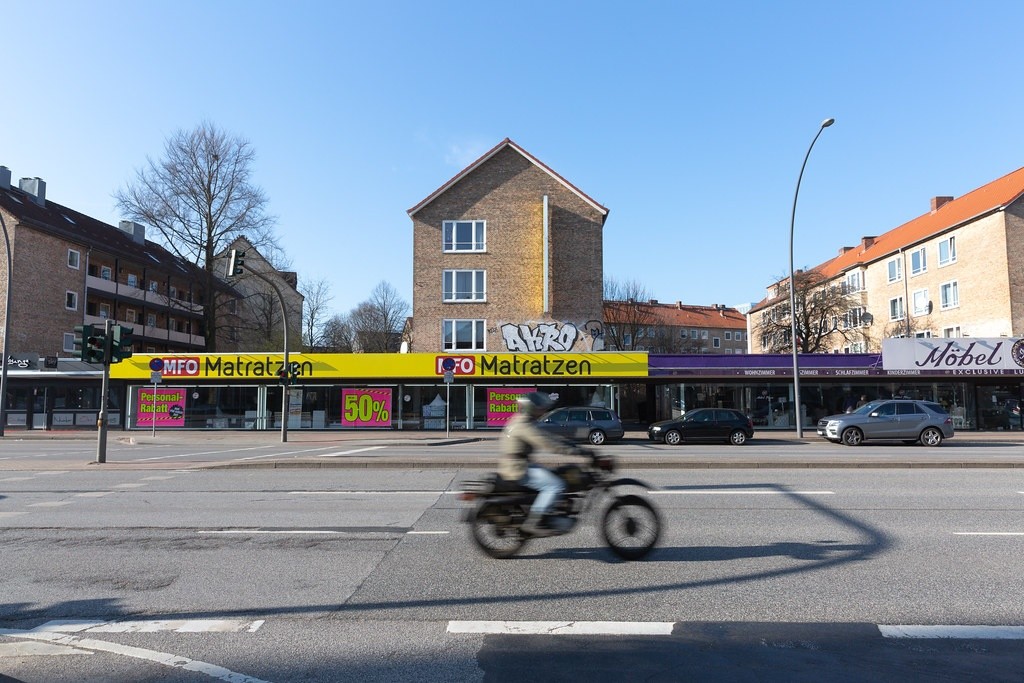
[278,370,287,386]
[72,325,92,362]
[89,336,111,368]
[228,249,245,277]
[112,325,134,363]
[290,362,300,385]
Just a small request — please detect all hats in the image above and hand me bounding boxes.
[527,392,553,411]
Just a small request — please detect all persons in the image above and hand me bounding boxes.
[856,395,868,409]
[497,392,592,537]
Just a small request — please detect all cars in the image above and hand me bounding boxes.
[648,408,754,446]
[533,405,624,445]
[816,398,954,448]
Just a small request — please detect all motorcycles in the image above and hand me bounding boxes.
[456,449,663,562]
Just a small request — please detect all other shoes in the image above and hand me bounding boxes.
[521,513,559,538]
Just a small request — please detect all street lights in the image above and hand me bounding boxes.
[788,117,837,438]
